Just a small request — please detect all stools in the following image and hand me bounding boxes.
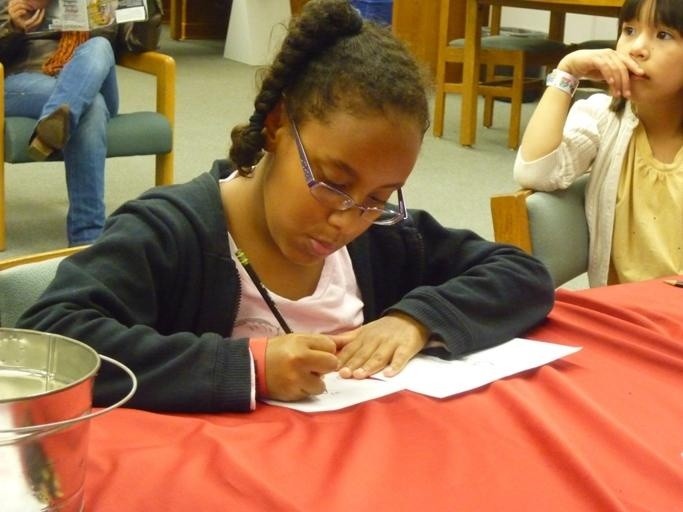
[0,42,179,248]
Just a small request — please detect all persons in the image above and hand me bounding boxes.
[0,0,167,248]
[25,8,47,31]
[11,0,557,415]
[513,0,682,290]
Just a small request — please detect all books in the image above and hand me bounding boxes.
[25,0,148,33]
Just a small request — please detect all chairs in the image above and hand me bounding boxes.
[486,171,594,294]
[430,0,564,153]
[0,245,91,330]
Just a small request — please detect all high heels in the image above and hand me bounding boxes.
[27,103,72,162]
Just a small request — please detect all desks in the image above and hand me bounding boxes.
[84,272,683,512]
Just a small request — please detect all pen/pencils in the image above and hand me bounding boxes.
[235,249,327,393]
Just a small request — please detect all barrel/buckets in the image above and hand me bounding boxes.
[0,326,137,512]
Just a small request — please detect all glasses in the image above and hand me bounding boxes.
[283,101,409,226]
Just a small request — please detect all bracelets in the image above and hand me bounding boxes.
[542,66,579,99]
